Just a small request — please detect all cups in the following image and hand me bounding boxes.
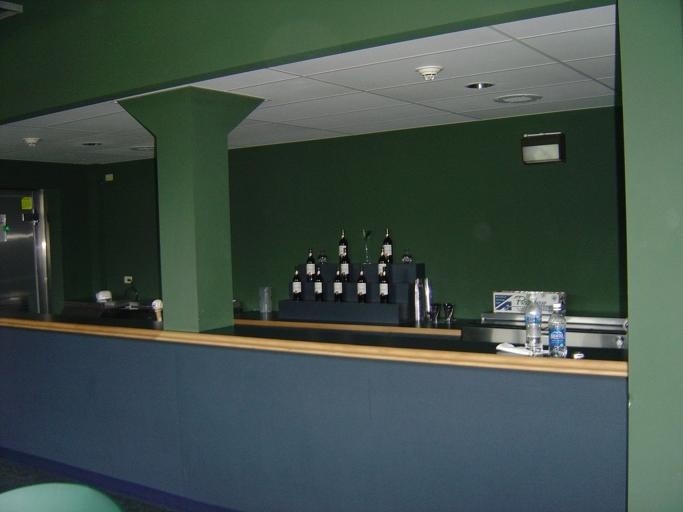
[258,286,273,314]
[431,304,456,324]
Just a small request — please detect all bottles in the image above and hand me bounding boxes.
[307,248,389,283]
[547,304,567,359]
[292,267,392,303]
[338,225,395,262]
[525,296,542,354]
